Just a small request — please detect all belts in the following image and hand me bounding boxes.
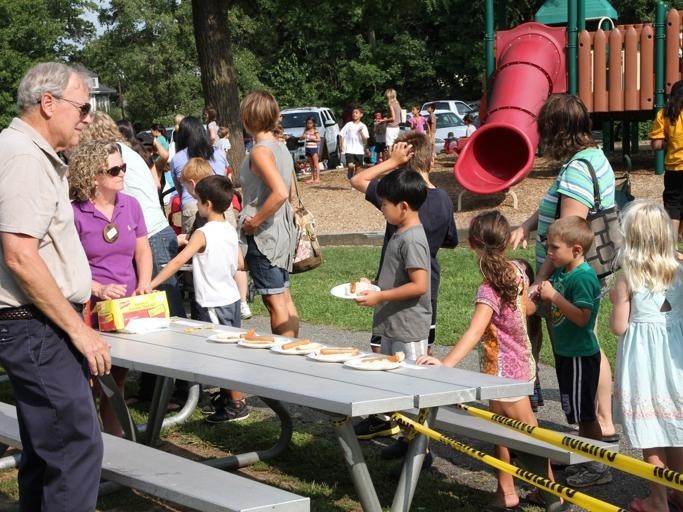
[1,304,87,320]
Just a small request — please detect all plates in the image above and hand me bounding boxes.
[160,261,194,272]
[327,281,380,300]
[206,329,401,371]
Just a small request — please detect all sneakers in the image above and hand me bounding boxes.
[565,463,612,488]
[201,393,225,414]
[206,399,250,423]
[354,415,401,439]
[381,438,434,469]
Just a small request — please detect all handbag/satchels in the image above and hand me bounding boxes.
[288,209,322,274]
[538,158,623,281]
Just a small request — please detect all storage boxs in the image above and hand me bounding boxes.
[96,285,169,335]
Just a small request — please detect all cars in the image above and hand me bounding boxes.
[404,111,475,154]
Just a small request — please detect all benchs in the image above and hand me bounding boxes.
[0,402,310,511]
[378,407,620,512]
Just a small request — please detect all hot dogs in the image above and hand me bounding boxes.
[345,281,366,296]
[216,327,405,367]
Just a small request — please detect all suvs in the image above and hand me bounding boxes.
[419,101,479,122]
[279,107,340,169]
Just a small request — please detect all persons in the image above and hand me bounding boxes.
[0,61,683,512]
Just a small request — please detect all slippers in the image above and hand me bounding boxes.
[628,496,647,512]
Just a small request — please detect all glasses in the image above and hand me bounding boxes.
[103,163,127,177]
[52,95,92,119]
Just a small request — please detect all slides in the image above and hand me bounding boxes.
[454,21,568,194]
